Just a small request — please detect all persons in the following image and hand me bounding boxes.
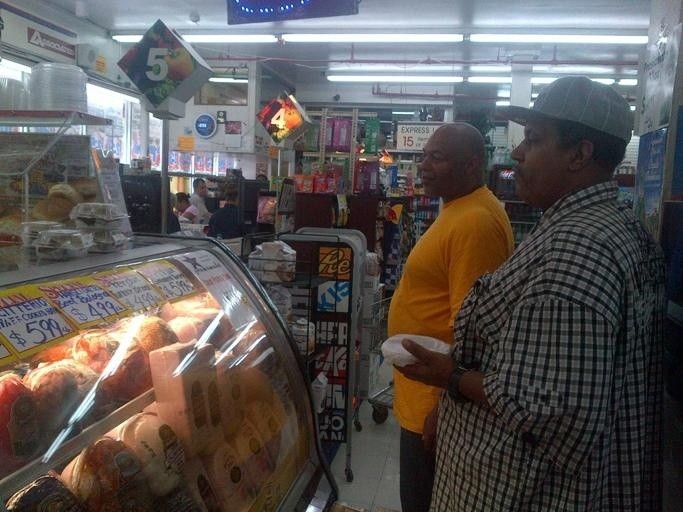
[388,122,515,512]
[393,75,666,512]
[167,173,267,239]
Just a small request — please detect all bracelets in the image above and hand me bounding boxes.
[448,367,472,402]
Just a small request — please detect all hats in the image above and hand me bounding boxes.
[496,76,633,143]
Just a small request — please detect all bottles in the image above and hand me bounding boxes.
[143,157,151,175]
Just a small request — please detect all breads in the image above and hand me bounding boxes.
[1,176,100,238]
[0,292,305,512]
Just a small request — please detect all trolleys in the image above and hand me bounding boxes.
[509,221,536,251]
[368,295,393,424]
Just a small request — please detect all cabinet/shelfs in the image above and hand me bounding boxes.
[491,165,544,245]
[0,108,370,512]
